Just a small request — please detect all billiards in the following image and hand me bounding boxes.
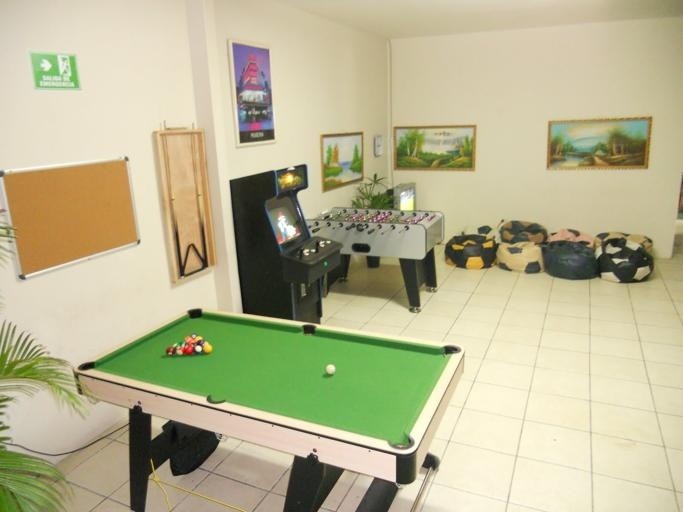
[326,365,336,374]
[166,333,213,356]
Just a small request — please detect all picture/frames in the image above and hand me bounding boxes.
[227,38,277,148]
[546,116,653,170]
[320,131,363,193]
[393,124,477,171]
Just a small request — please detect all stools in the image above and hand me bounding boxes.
[444,220,655,284]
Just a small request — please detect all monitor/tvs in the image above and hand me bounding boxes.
[266,196,302,246]
[371,182,416,211]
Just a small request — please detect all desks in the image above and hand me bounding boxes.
[72,306,465,512]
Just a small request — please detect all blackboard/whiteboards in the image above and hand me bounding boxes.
[1,155,141,281]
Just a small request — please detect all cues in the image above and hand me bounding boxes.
[161,117,207,276]
